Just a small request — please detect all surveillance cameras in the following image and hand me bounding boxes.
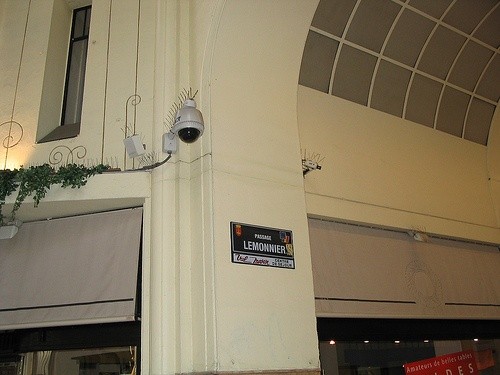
[302,159,321,171]
[175,100,204,144]
